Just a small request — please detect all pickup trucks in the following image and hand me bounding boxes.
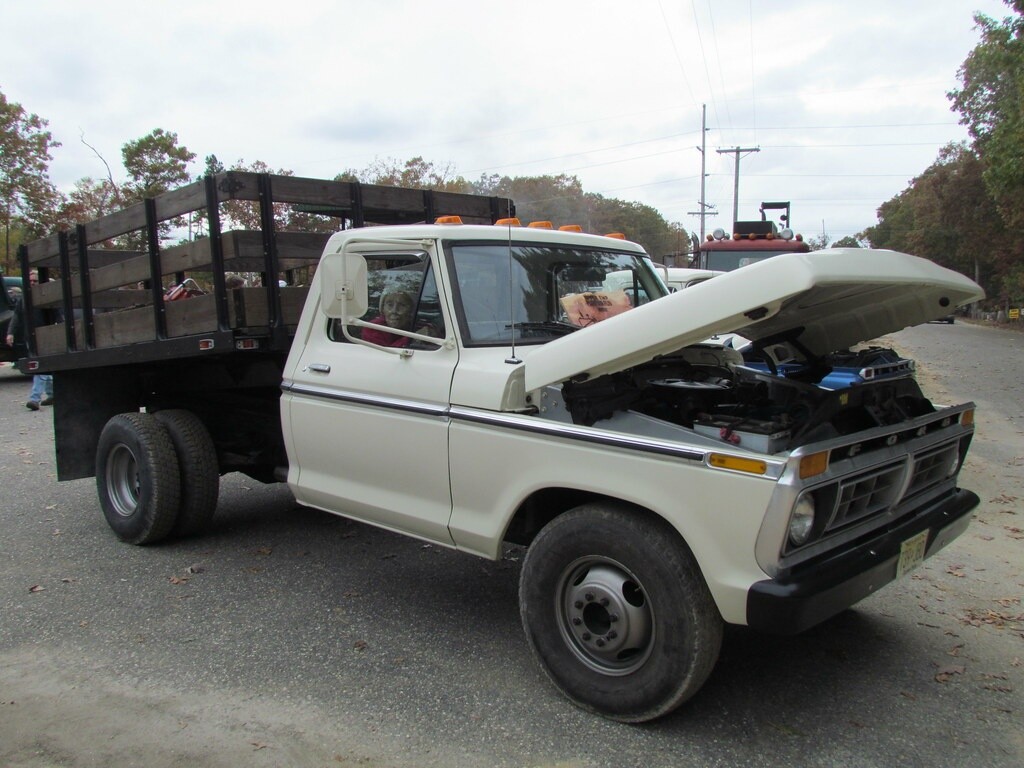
[597,266,727,307]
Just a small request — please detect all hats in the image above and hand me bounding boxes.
[379,282,417,315]
[8,287,22,293]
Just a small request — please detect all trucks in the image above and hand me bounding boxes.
[18,168,987,723]
[692,221,811,274]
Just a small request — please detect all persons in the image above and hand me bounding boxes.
[227,276,244,288]
[6,269,63,410]
[138,282,143,289]
[362,281,437,349]
[169,281,176,292]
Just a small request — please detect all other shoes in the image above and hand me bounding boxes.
[41,396,55,405]
[26,401,40,410]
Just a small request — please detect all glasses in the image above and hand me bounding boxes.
[29,280,38,282]
